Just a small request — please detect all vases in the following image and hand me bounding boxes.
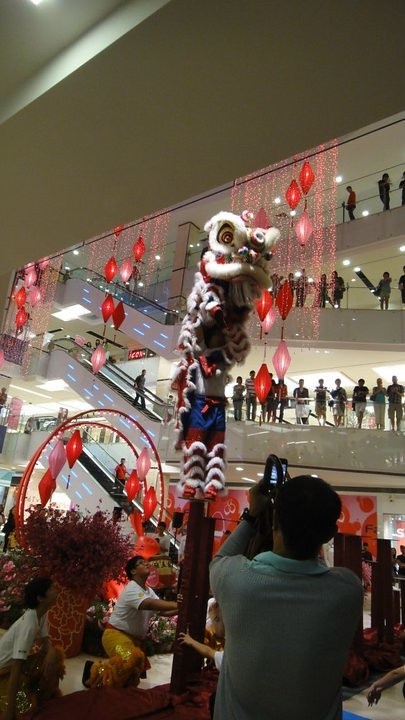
[0,566,176,658]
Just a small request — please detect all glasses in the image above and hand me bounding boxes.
[135,560,147,568]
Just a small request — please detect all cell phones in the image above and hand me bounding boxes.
[269,458,288,492]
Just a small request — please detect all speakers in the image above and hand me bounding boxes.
[172,512,184,528]
[113,507,122,522]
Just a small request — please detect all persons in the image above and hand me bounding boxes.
[360,541,405,589]
[0,502,188,600]
[131,369,147,410]
[209,474,366,720]
[231,369,311,425]
[351,379,370,430]
[386,376,405,432]
[372,378,387,433]
[112,458,128,501]
[0,574,66,720]
[329,379,347,430]
[366,663,405,707]
[81,555,179,690]
[176,623,226,720]
[266,170,405,310]
[0,265,142,436]
[314,379,330,427]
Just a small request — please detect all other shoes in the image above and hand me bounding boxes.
[82,660,95,689]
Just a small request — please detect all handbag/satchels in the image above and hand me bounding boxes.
[296,402,311,418]
[370,395,376,401]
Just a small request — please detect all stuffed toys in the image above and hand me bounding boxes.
[166,209,281,502]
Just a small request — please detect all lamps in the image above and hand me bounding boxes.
[8,255,49,329]
[38,430,160,561]
[252,158,314,405]
[90,214,147,375]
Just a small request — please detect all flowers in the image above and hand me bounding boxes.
[0,504,179,645]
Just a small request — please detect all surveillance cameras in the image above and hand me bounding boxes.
[389,497,394,502]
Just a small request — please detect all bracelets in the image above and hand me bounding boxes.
[240,507,264,532]
